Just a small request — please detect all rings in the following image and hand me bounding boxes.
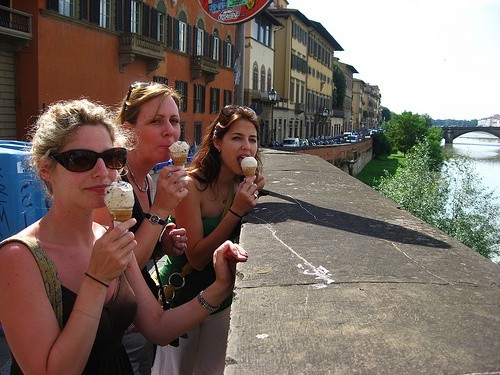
[253,193,258,198]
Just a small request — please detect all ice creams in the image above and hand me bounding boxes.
[169,141,190,167]
[241,156,258,181]
[104,180,134,229]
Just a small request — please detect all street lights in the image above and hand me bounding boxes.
[322,107,330,145]
[268,89,278,149]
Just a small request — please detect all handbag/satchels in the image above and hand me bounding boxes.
[158,303,190,347]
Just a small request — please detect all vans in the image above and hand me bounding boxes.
[282,137,300,148]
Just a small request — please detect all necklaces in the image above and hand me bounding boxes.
[127,165,148,192]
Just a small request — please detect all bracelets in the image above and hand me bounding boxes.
[198,291,220,312]
[228,208,241,219]
[85,272,109,287]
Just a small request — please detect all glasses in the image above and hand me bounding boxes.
[124,81,169,109]
[47,147,127,173]
[218,105,257,126]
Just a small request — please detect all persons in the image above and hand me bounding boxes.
[137,103,264,375]
[0,97,248,375]
[92,82,187,375]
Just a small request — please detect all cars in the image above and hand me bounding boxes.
[299,127,383,146]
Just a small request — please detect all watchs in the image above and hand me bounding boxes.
[143,213,166,226]
